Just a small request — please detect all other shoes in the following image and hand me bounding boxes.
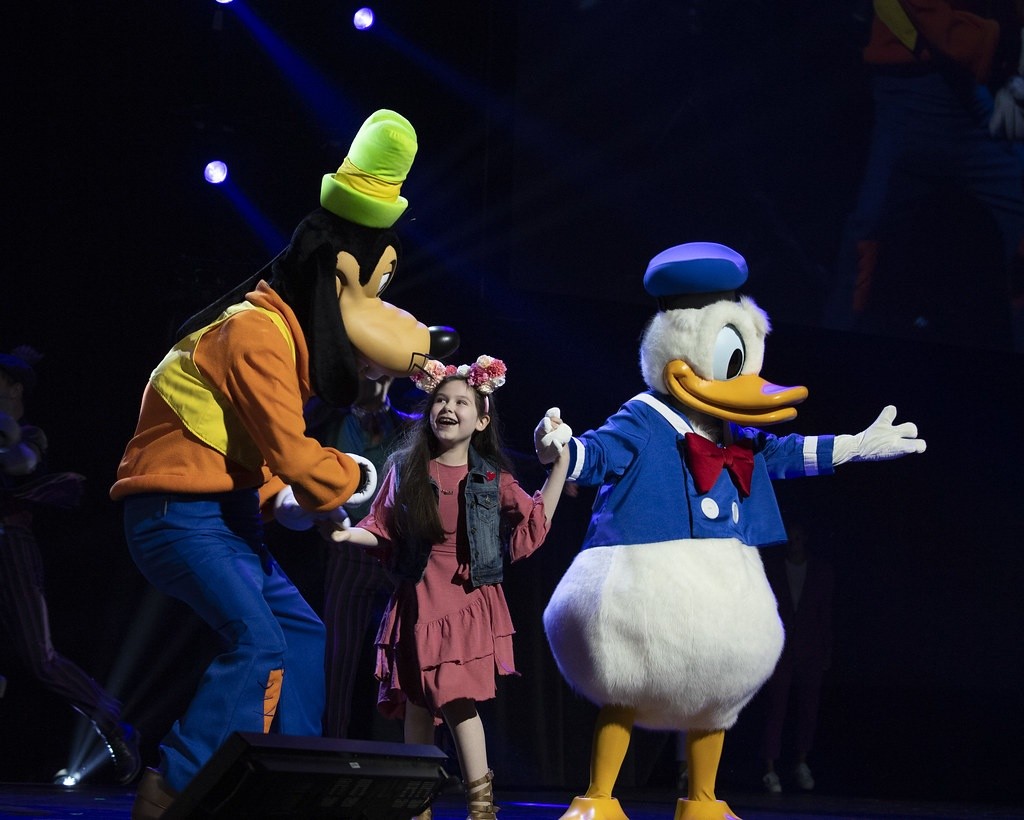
[411,796,431,820]
[461,769,500,820]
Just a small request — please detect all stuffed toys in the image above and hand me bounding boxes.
[110,109,460,820]
[532,241,928,820]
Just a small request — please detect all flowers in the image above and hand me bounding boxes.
[410,354,507,393]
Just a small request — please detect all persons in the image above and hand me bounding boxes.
[317,363,411,743]
[318,355,571,820]
[0,355,142,785]
[819,0,1024,354]
[764,523,835,794]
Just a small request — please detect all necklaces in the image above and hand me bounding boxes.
[436,458,466,495]
